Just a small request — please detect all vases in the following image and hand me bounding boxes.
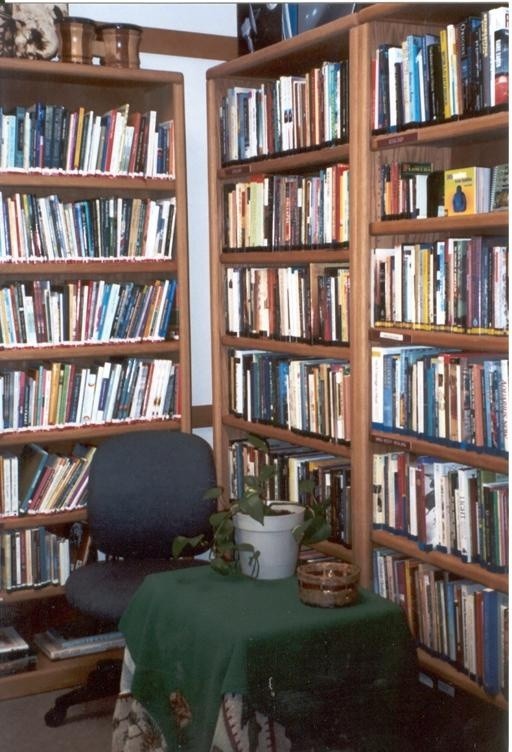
[228,501,307,584]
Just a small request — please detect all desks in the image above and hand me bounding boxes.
[110,563,409,752]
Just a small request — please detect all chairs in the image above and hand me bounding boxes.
[45,430,216,727]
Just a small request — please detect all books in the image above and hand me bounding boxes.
[370,545,510,705]
[368,453,512,575]
[369,343,509,454]
[2,520,125,594]
[379,159,509,219]
[0,617,128,677]
[0,357,179,429]
[369,5,507,133]
[225,167,349,248]
[0,443,101,515]
[225,350,351,444]
[0,189,176,260]
[0,275,177,343]
[369,236,509,333]
[218,57,349,163]
[0,101,177,177]
[224,261,350,345]
[223,436,357,574]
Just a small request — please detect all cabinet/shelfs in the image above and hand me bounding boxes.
[0,58,192,705]
[356,2,510,721]
[206,11,356,569]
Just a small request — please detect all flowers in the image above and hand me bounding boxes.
[171,432,339,581]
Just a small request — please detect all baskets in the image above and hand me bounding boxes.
[297,561,362,608]
